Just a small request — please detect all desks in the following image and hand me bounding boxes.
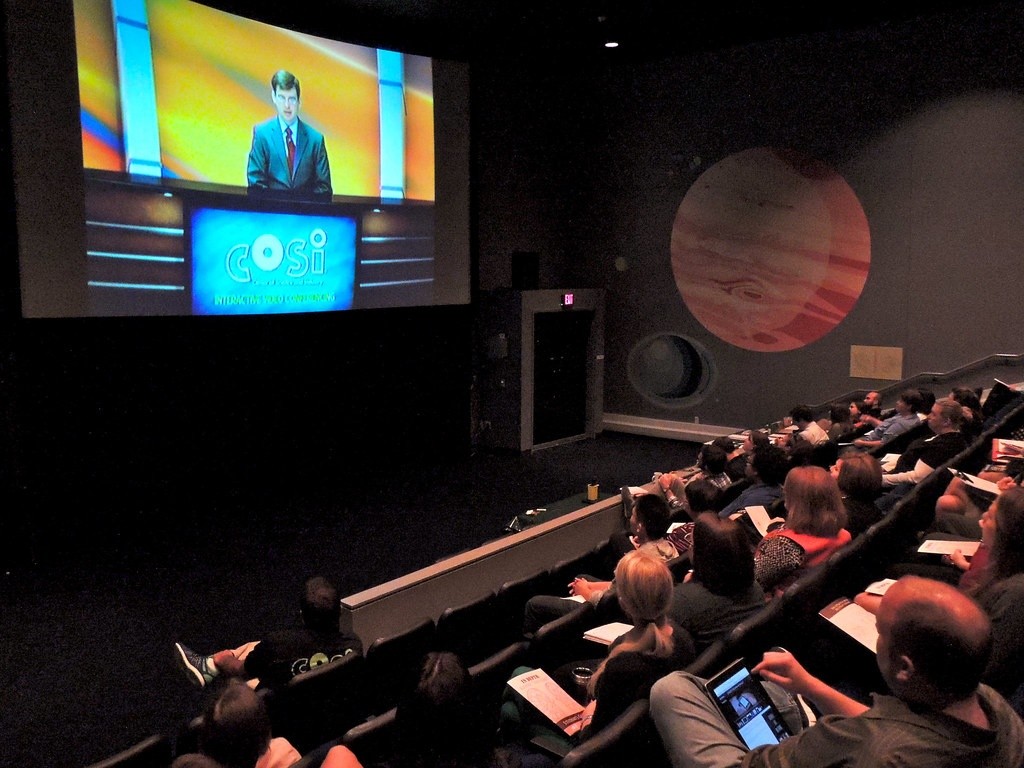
[519,492,613,525]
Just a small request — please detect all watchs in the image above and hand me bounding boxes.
[662,487,671,494]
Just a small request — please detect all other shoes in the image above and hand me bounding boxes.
[622,484,635,519]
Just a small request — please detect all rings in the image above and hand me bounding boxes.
[949,561,955,566]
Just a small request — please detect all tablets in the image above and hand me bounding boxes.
[705,657,794,752]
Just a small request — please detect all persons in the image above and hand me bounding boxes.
[245,70,333,198]
[170,372,1024,768]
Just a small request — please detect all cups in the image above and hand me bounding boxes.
[652,471,662,484]
[571,667,593,708]
[588,483,599,500]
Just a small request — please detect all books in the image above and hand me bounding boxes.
[583,622,634,646]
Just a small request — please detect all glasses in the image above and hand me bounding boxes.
[850,406,858,410]
[746,459,753,466]
[866,397,875,401]
[275,94,298,106]
[748,436,754,447]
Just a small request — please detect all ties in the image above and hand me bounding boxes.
[285,127,295,179]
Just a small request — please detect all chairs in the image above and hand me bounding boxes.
[95,380,1024,768]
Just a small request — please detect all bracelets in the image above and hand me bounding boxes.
[580,714,592,731]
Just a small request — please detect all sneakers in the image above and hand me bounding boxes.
[173,641,217,691]
